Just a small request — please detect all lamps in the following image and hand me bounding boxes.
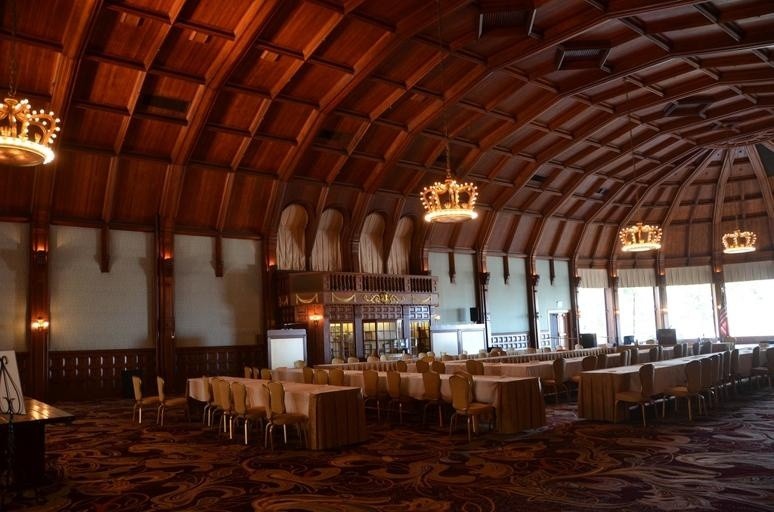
[533,274,539,286]
[576,277,581,287]
[269,265,278,273]
[165,259,173,265]
[658,275,666,285]
[722,135,758,254]
[37,251,45,257]
[619,76,664,253]
[419,0,479,223]
[482,271,490,285]
[712,272,722,281]
[613,276,619,287]
[0,0,61,167]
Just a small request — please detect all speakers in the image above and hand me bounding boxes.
[470,307,479,321]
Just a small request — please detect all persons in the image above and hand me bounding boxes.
[344,347,350,363]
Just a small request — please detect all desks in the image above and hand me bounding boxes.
[0,394,76,491]
